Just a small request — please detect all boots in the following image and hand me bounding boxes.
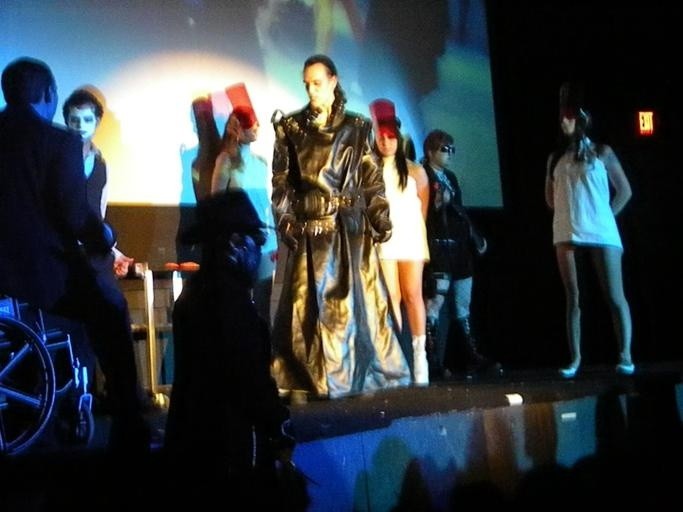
[424,316,453,381]
[457,315,503,377]
[411,335,429,388]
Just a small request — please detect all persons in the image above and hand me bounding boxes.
[368,98,432,387]
[209,105,274,323]
[60,88,134,288]
[544,98,634,381]
[271,53,415,403]
[1,55,165,453]
[421,129,505,381]
[158,188,311,512]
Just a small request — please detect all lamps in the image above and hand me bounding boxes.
[638,111,653,134]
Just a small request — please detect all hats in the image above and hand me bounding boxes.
[196,189,267,233]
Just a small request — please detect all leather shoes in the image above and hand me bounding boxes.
[558,365,579,378]
[615,363,636,375]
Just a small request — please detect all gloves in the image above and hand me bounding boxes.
[371,219,392,243]
[279,219,301,253]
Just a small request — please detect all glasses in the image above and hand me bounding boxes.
[439,144,455,154]
